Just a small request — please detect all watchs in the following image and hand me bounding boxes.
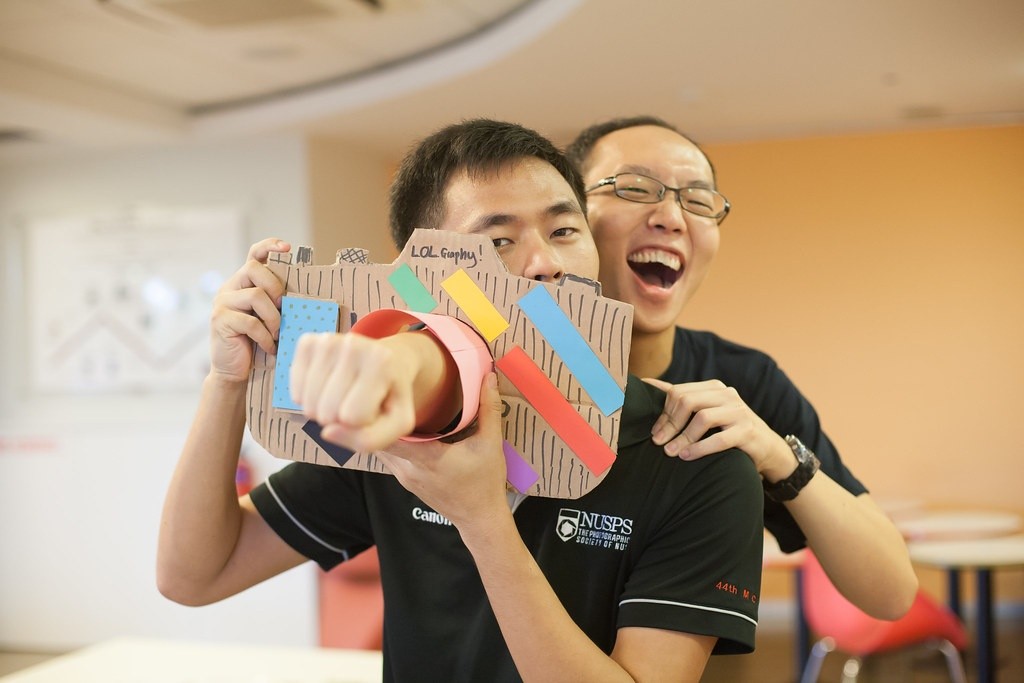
[766,434,821,502]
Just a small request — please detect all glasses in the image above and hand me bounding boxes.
[584,173,730,226]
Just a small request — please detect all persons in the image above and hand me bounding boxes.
[156,122,762,683]
[288,116,918,619]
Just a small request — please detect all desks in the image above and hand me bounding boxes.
[758,506,1024,682]
